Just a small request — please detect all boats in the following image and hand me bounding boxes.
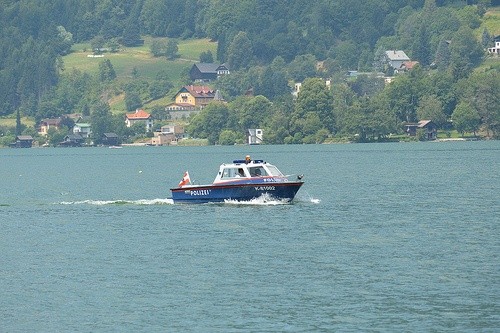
[170,159,305,207]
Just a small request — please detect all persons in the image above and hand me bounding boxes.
[235,168,245,178]
[253,169,263,177]
